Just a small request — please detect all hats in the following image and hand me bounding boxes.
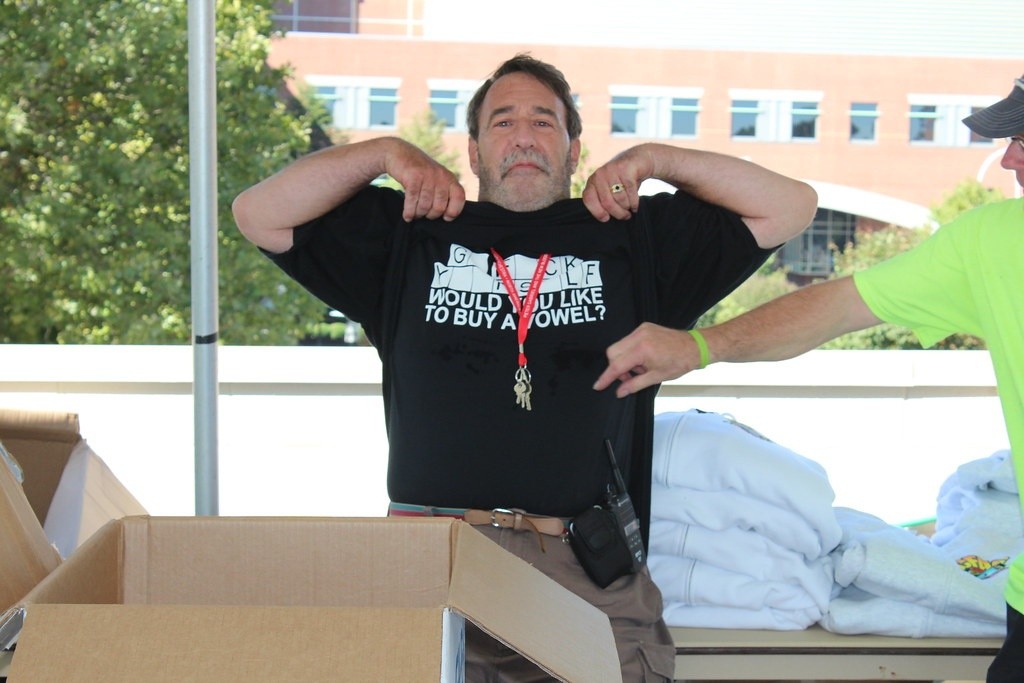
[961,73,1024,138]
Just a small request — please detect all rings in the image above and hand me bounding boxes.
[611,183,624,194]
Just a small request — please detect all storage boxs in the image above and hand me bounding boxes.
[0,406,627,683]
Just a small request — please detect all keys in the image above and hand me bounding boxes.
[514,381,532,411]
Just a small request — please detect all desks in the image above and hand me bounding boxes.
[665,622,1013,683]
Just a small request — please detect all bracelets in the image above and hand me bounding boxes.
[687,329,708,369]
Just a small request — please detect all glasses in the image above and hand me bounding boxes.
[1011,137,1024,149]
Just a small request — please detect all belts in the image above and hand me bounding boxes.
[388,502,572,555]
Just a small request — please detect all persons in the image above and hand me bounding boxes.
[232,59,817,683]
[595,74,1024,683]
